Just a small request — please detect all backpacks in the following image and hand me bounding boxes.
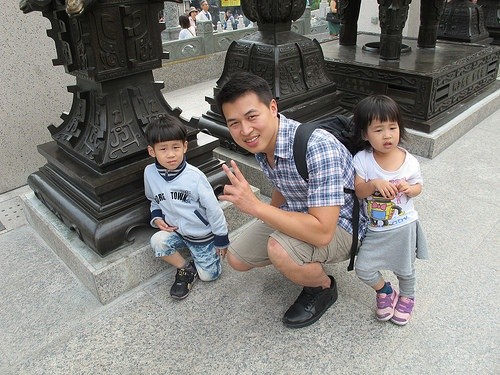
[293,114,367,194]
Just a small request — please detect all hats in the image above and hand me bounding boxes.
[188,6,198,16]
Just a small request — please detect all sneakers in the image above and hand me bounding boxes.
[376,280,399,322]
[390,295,415,325]
[170,260,199,299]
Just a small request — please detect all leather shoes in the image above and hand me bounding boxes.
[282,275,338,328]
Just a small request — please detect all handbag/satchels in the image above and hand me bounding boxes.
[326,13,340,23]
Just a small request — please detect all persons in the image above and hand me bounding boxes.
[216,72,369,329]
[351,94,430,327]
[179,0,215,40]
[224,11,239,31]
[143,114,230,300]
[326,0,341,38]
